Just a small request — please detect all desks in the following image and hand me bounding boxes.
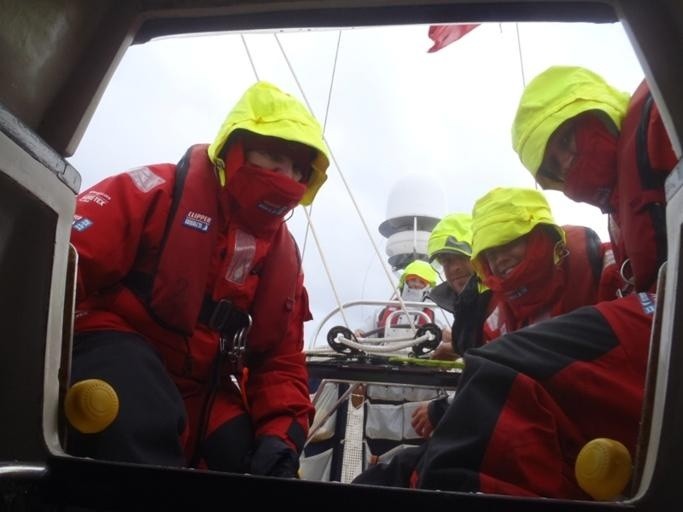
[303,348,465,482]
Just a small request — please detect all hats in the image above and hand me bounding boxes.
[469,187,567,276]
[511,65,631,191]
[427,213,490,295]
[399,260,436,291]
[208,80,330,206]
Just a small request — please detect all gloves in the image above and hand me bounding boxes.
[248,434,299,480]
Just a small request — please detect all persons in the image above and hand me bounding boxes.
[349,258,462,466]
[350,186,637,489]
[413,213,510,359]
[410,65,678,502]
[57,80,331,478]
